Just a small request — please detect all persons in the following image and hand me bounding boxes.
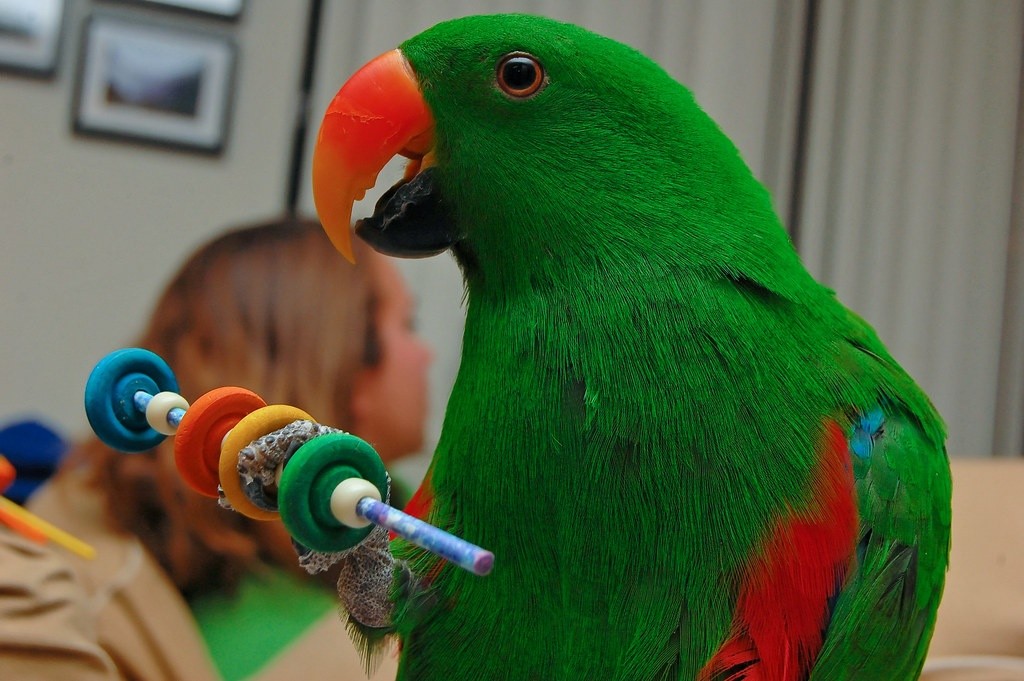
[25,220,433,677]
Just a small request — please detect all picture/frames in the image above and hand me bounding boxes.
[74,10,241,159]
[0,0,68,79]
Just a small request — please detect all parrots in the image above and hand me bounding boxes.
[217,14,951,681]
